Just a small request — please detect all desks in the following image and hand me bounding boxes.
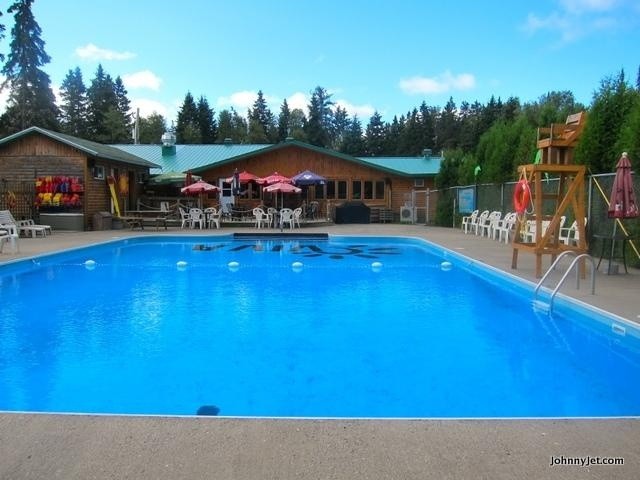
[591,233,637,275]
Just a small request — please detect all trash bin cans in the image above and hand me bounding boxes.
[93,211,112,230]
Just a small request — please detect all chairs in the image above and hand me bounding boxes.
[536,110,585,150]
[461,208,588,249]
[0,209,52,253]
[179,200,319,232]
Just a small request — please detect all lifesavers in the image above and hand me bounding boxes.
[514,180,529,214]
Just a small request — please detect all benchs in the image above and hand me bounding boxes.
[119,217,167,232]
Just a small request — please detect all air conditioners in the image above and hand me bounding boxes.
[399,205,418,222]
[411,178,424,187]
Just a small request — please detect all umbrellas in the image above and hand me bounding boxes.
[180,179,221,210]
[608,151,639,261]
[263,182,302,210]
[225,171,264,185]
[257,171,292,208]
[291,169,328,209]
[232,167,241,209]
